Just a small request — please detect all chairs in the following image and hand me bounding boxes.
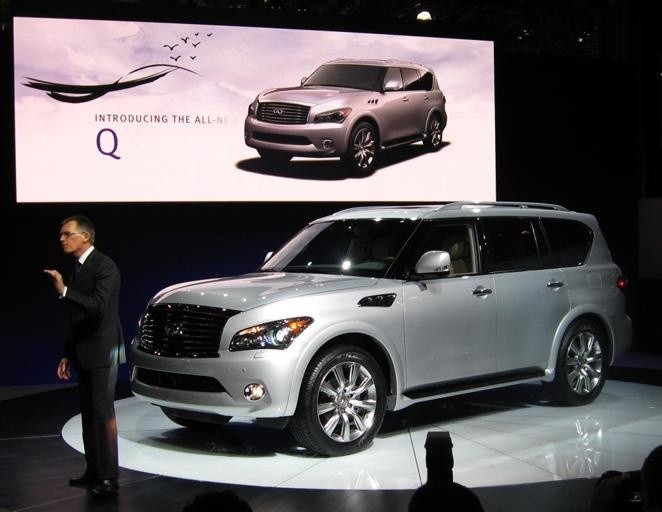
[358,230,395,270]
[436,237,471,275]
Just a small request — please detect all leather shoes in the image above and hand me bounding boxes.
[68,474,118,498]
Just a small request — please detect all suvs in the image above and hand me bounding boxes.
[242,58,450,176]
[128,200,635,454]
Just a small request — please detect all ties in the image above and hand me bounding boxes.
[73,260,79,272]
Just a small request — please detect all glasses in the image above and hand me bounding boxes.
[57,231,86,238]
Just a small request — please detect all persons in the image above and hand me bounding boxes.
[183,488,253,511]
[44,216,127,496]
[408,481,484,511]
